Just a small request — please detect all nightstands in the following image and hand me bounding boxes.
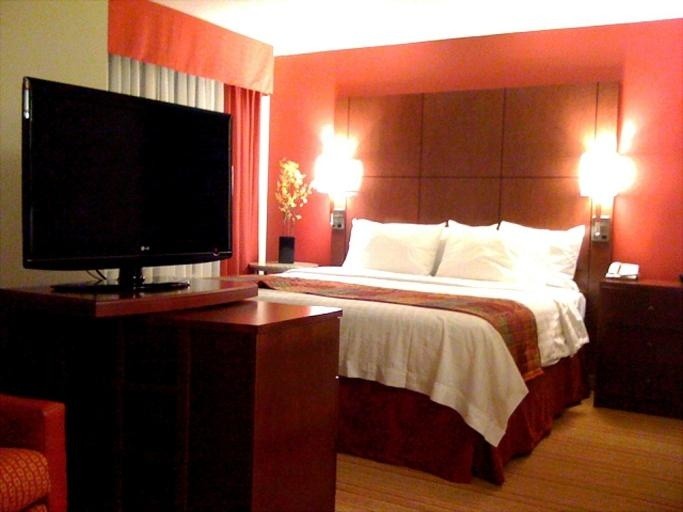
[248,261,318,275]
[594,278,683,419]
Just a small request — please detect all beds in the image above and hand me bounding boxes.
[205,267,597,484]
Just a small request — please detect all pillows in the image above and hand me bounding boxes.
[342,217,585,282]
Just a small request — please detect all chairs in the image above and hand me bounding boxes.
[0,395,68,512]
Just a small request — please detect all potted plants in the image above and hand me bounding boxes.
[276,160,312,264]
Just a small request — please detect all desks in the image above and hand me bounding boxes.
[0,275,343,512]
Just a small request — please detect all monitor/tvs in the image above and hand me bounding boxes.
[19,75,232,293]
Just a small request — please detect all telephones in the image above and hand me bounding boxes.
[606,261,640,280]
